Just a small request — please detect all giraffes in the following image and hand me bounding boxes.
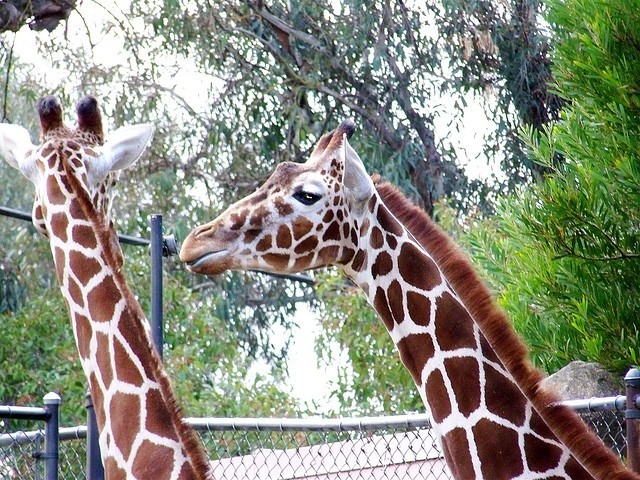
[179,115,640,480]
[0,92,215,480]
